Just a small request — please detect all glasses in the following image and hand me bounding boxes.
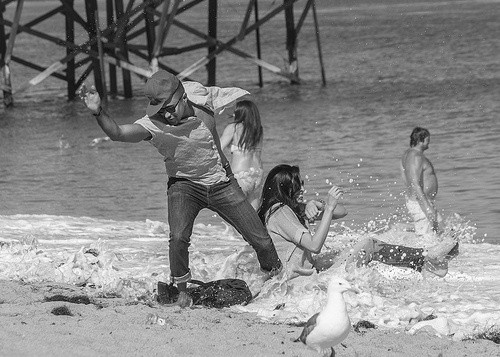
[157,94,183,113]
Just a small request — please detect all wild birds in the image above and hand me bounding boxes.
[289,278,360,357]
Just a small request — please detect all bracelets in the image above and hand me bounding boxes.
[317,199,326,210]
[93,106,103,116]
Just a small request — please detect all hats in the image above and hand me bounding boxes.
[144,69,180,117]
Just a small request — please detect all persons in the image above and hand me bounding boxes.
[258,163,460,279]
[218,101,265,212]
[79,71,284,308]
[400,126,439,240]
